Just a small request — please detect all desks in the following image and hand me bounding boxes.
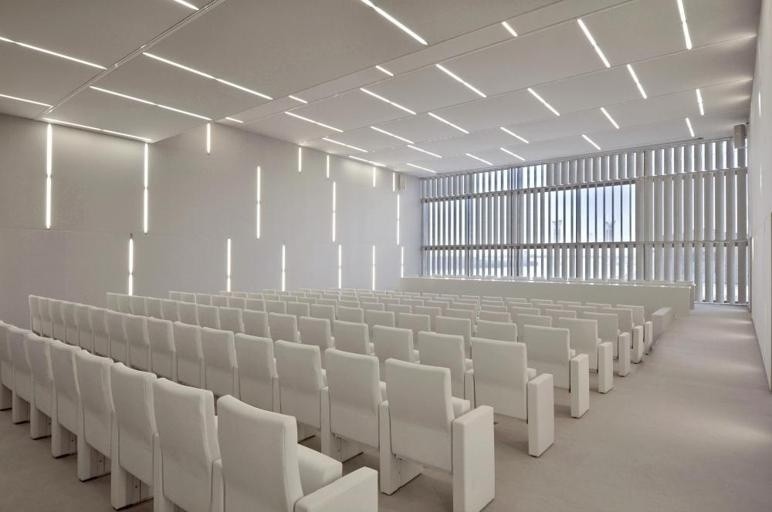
[402,277,691,315]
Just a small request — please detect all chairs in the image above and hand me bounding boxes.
[452,303,476,308]
[197,304,218,328]
[601,307,643,365]
[228,297,246,311]
[331,320,375,358]
[321,347,423,496]
[569,305,595,313]
[617,305,653,355]
[299,297,318,303]
[8,327,51,440]
[152,378,224,512]
[162,298,178,321]
[388,304,412,313]
[483,296,503,300]
[242,309,268,340]
[247,293,262,299]
[523,324,590,420]
[379,298,400,304]
[62,301,79,345]
[504,297,528,302]
[109,361,164,512]
[264,294,278,300]
[129,295,145,316]
[378,357,495,512]
[481,300,503,305]
[457,298,478,303]
[529,298,553,307]
[368,324,420,383]
[145,297,162,319]
[211,395,377,511]
[360,297,378,303]
[587,302,611,308]
[172,321,206,389]
[195,294,211,305]
[90,308,111,355]
[76,352,153,512]
[481,304,508,312]
[546,309,575,319]
[365,310,395,343]
[310,303,335,337]
[316,299,337,305]
[446,308,476,333]
[557,317,615,394]
[218,306,241,332]
[268,311,297,343]
[426,301,449,312]
[335,305,364,324]
[287,302,309,316]
[106,292,117,311]
[435,316,471,358]
[49,299,68,343]
[558,301,581,307]
[116,293,129,313]
[507,302,532,309]
[479,310,511,322]
[27,334,77,459]
[233,293,245,298]
[199,327,239,399]
[178,301,197,326]
[38,297,56,339]
[539,304,562,310]
[340,301,360,306]
[582,311,630,377]
[76,304,94,354]
[252,288,439,299]
[106,311,130,364]
[265,300,286,314]
[516,314,553,344]
[412,329,476,411]
[280,296,296,302]
[49,340,110,485]
[123,313,151,372]
[397,312,431,350]
[0,320,30,425]
[462,295,479,298]
[28,294,42,336]
[145,316,178,383]
[401,299,424,305]
[440,294,458,300]
[210,295,229,307]
[181,292,197,303]
[464,337,554,459]
[168,291,181,302]
[476,320,518,342]
[360,302,384,311]
[232,332,317,446]
[297,316,333,369]
[246,299,265,312]
[272,339,365,464]
[414,306,442,331]
[511,307,539,315]
[436,297,455,304]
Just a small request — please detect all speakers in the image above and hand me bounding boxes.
[734,124,745,147]
